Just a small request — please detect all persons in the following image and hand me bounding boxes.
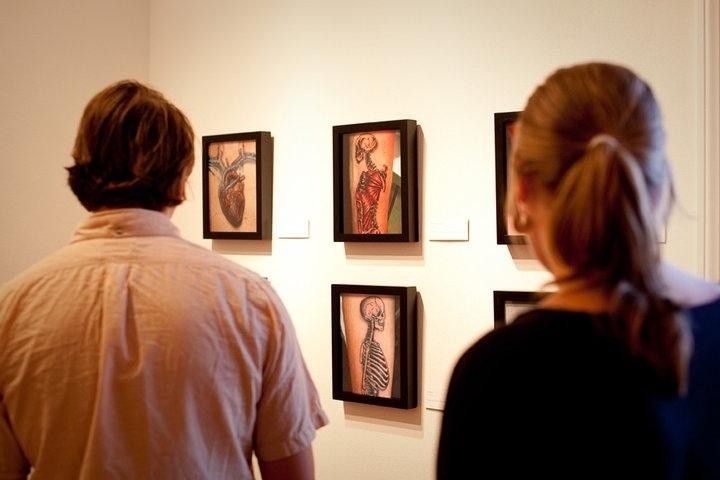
[435,60,719,477]
[1,78,330,478]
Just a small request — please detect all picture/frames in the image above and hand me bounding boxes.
[495,110,557,331]
